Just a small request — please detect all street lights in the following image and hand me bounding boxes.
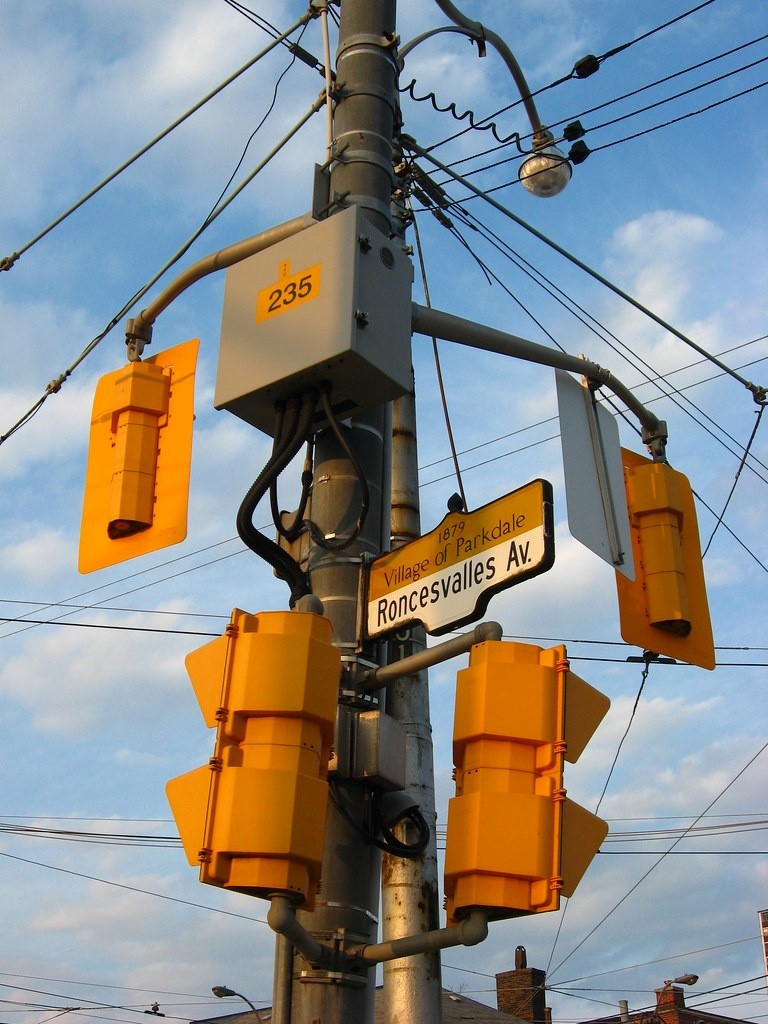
[272,1,572,1024]
